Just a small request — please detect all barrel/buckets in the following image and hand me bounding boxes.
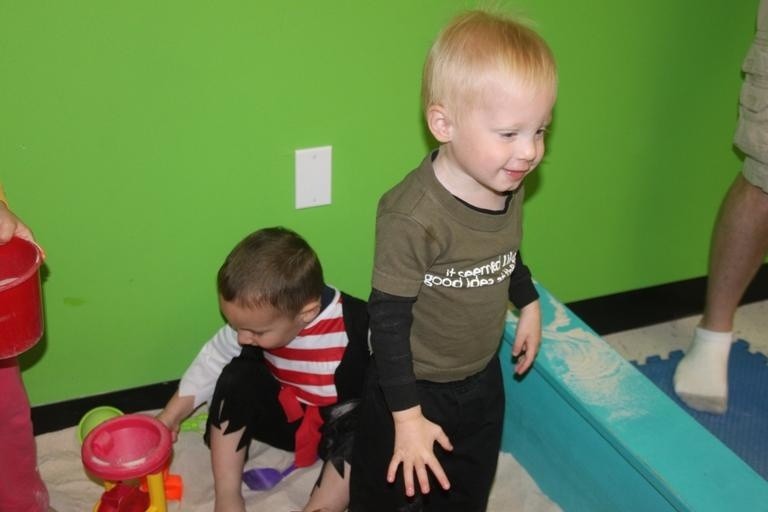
[0,236,45,359]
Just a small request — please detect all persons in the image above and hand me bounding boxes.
[349,10,558,511]
[671,0,768,416]
[153,226,368,512]
[0,180,54,512]
